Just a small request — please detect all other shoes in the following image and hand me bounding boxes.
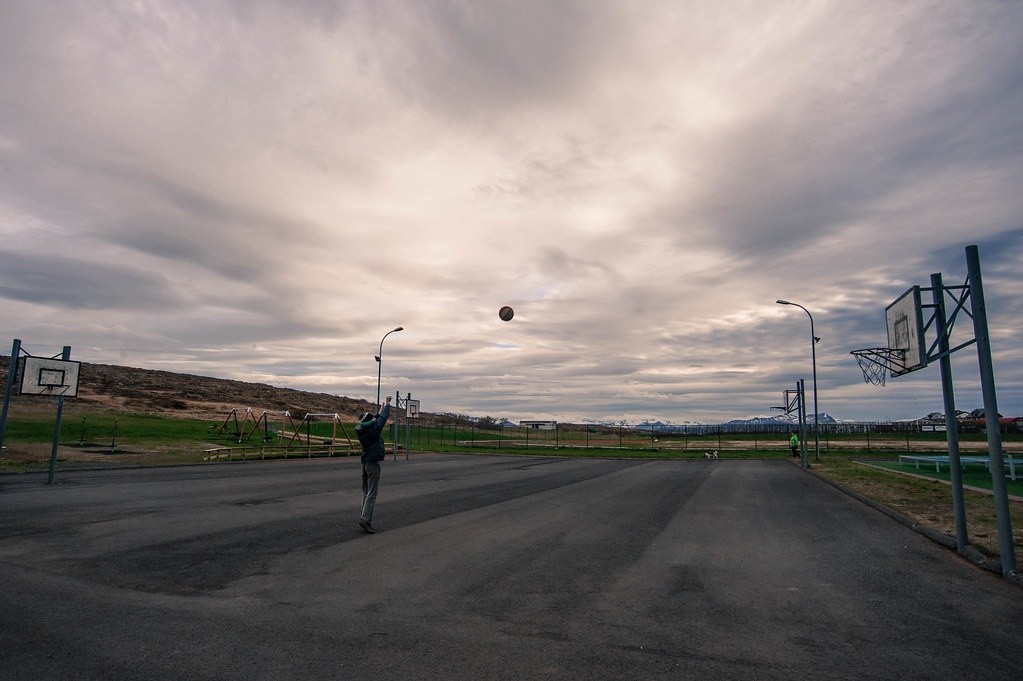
[358,520,376,533]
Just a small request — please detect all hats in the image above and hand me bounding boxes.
[358,412,373,422]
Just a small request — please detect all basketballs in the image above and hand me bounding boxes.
[499,305,514,322]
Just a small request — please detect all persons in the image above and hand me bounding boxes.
[354,395,392,533]
[790,430,801,460]
